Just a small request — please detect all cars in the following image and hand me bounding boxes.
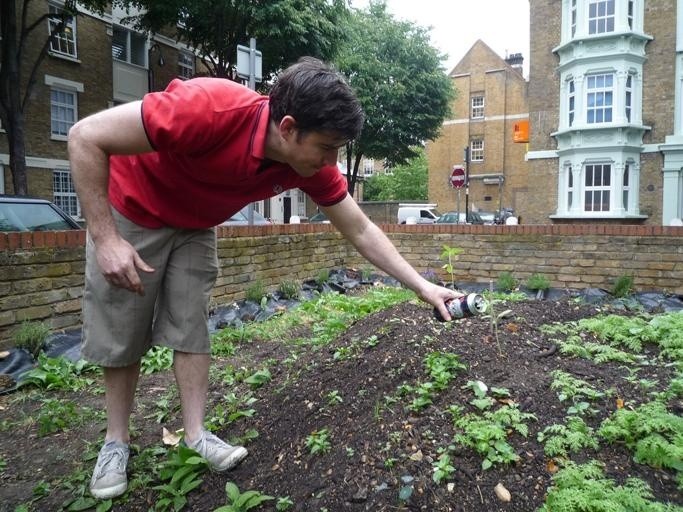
[216,204,273,227]
[305,212,330,223]
[0,193,84,232]
[397,201,517,226]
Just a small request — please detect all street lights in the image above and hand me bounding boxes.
[144,42,165,93]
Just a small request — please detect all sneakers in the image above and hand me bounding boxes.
[184,428,249,473]
[89,436,130,500]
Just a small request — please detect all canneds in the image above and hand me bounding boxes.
[433,292,487,322]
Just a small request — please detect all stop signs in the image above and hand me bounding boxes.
[450,167,466,188]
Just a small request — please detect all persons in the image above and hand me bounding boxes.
[62,56,470,501]
